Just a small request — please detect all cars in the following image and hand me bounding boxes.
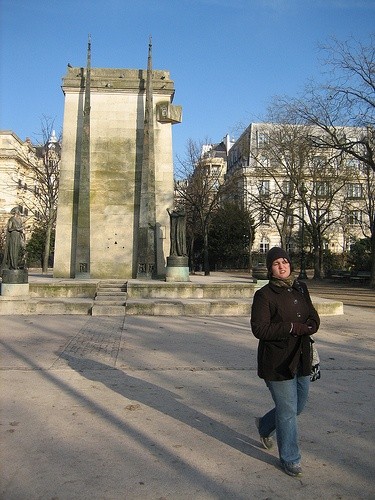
[253,262,272,279]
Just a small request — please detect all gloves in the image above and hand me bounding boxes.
[306,319,318,337]
[289,323,310,337]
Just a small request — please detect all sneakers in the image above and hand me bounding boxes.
[281,456,302,477]
[254,416,273,450]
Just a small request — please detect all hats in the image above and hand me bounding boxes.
[266,247,290,270]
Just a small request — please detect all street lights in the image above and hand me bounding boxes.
[298,182,307,278]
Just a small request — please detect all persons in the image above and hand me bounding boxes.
[3,207,29,269]
[250,246,320,475]
